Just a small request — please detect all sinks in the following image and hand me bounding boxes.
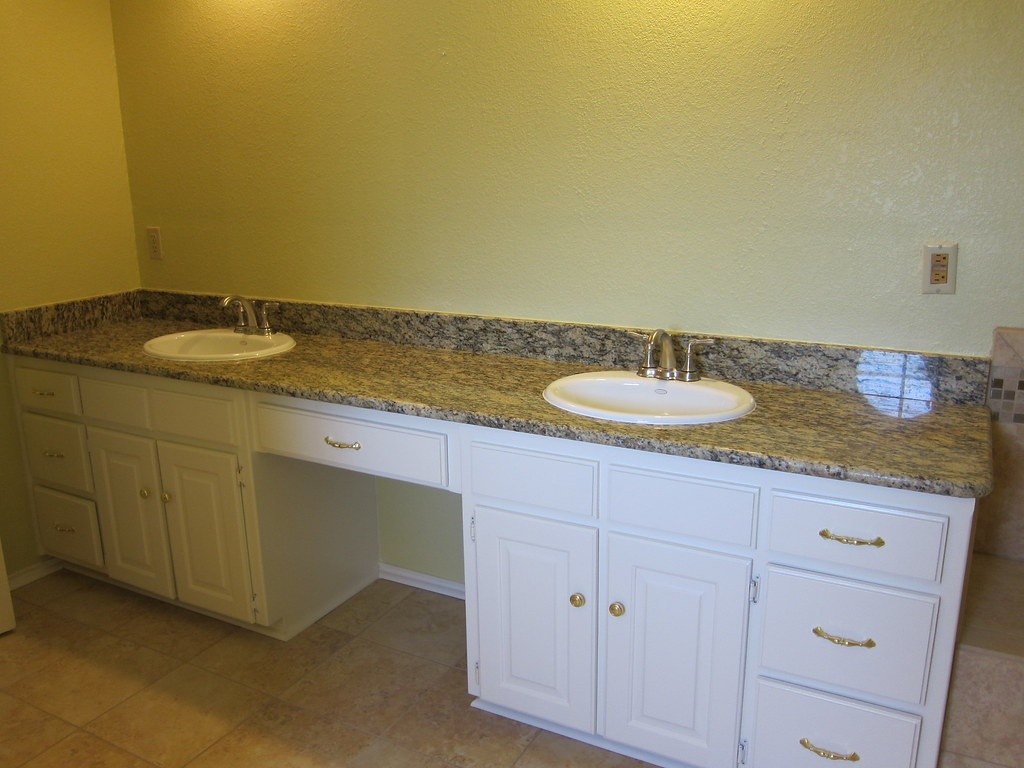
[541,367,757,425]
[141,326,297,362]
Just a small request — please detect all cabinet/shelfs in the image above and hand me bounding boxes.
[245,390,462,496]
[6,353,109,583]
[737,470,975,768]
[461,423,761,768]
[78,365,380,642]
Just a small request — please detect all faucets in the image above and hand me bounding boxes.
[217,295,259,335]
[647,328,678,380]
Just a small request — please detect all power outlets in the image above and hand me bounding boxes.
[922,243,958,294]
[146,227,163,262]
